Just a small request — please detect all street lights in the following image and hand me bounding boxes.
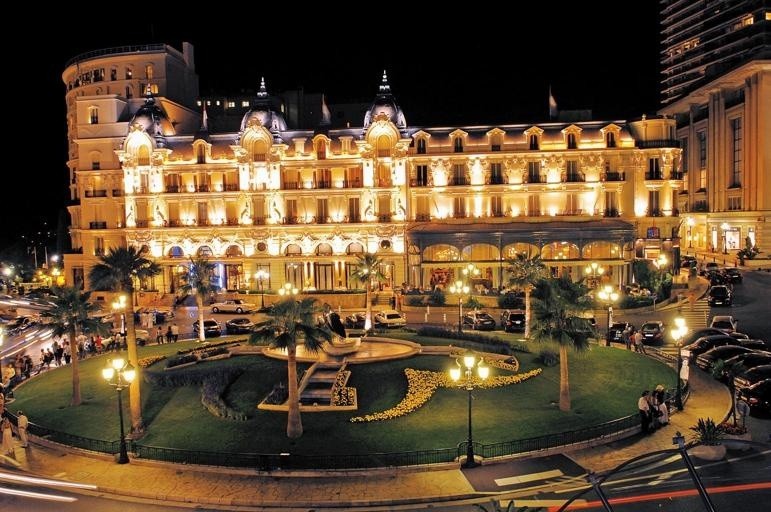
[720,222,730,255]
[670,314,692,412]
[585,261,605,308]
[653,253,668,280]
[449,280,471,332]
[687,217,694,248]
[277,282,298,300]
[112,294,127,350]
[51,268,59,281]
[447,344,493,469]
[100,351,139,466]
[598,285,621,347]
[253,269,272,313]
[462,261,481,295]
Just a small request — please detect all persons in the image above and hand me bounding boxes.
[165,326,172,343]
[622,327,647,355]
[141,308,158,329]
[17,410,29,448]
[638,385,669,435]
[1,417,14,454]
[171,323,179,343]
[0,331,121,415]
[156,326,163,344]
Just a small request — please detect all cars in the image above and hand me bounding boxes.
[638,319,667,346]
[83,326,150,353]
[192,317,223,338]
[464,309,497,331]
[134,306,176,325]
[707,284,735,306]
[710,313,741,333]
[225,317,256,334]
[680,253,747,285]
[684,327,770,415]
[374,309,408,328]
[609,321,637,344]
[209,298,256,314]
[0,283,53,338]
[345,311,366,328]
[400,282,537,296]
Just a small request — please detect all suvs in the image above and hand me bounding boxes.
[499,308,526,333]
